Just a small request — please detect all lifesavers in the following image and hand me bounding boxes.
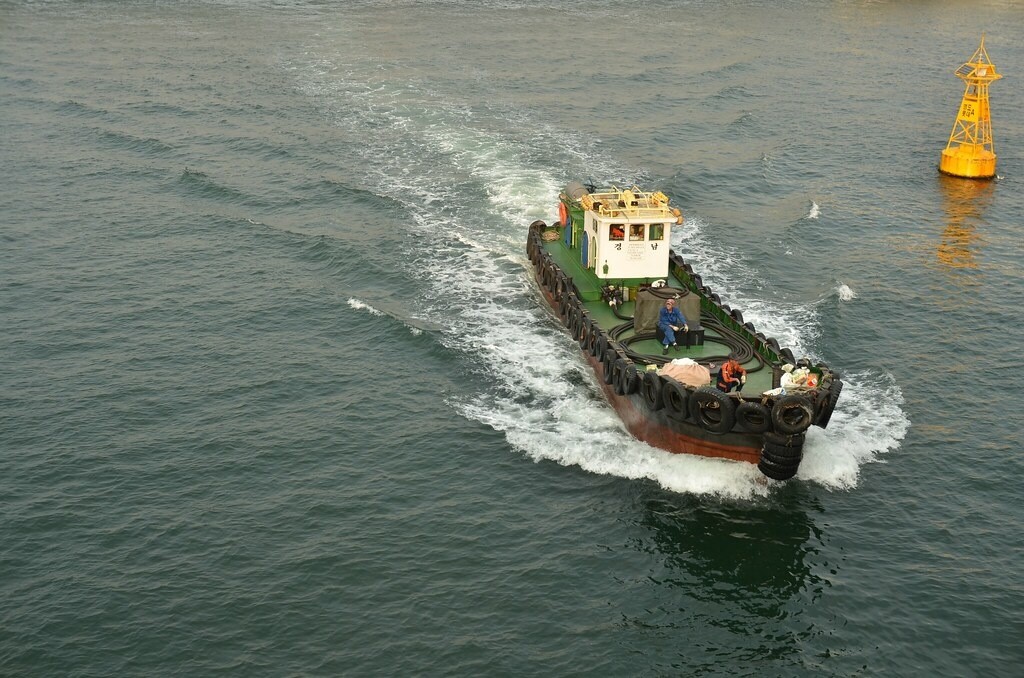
[643,372,661,412]
[596,337,608,362]
[578,318,590,350]
[565,301,577,328]
[661,380,689,421]
[613,359,627,395]
[558,203,567,227]
[734,402,771,432]
[623,365,637,396]
[669,247,842,429]
[570,309,583,341]
[689,387,734,435]
[603,349,617,384]
[588,329,601,357]
[758,396,811,480]
[526,232,569,316]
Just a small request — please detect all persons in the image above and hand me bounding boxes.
[716,353,747,393]
[655,299,689,355]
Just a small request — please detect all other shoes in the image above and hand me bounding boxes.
[673,343,680,351]
[663,348,669,355]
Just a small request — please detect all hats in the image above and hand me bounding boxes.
[667,299,675,306]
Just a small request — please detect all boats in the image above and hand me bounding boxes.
[525,178,843,483]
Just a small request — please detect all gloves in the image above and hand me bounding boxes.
[741,376,746,384]
[673,326,679,332]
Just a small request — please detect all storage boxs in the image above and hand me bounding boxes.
[654,319,705,348]
